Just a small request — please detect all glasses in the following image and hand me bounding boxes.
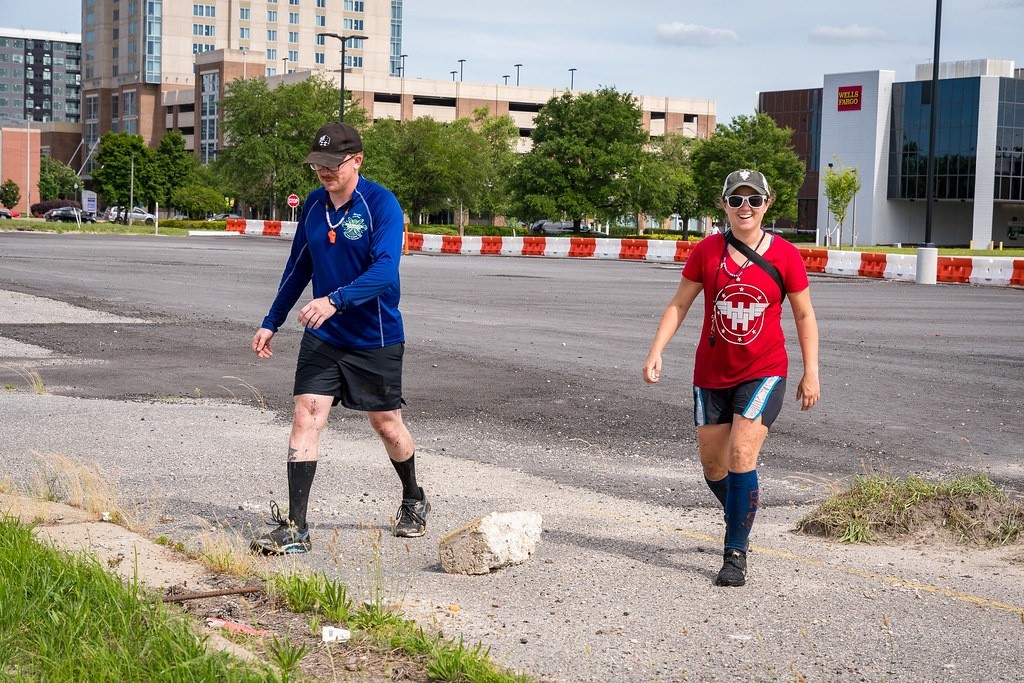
[309,153,359,172]
[723,193,769,208]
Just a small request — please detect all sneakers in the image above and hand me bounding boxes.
[394,487,431,538]
[716,548,748,587]
[723,515,749,551]
[251,500,312,554]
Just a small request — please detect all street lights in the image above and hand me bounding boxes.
[458,59,467,81]
[851,170,856,246]
[514,63,524,86]
[73,183,79,202]
[396,67,403,77]
[568,68,577,90]
[317,33,370,123]
[449,71,458,81]
[281,57,289,74]
[400,54,408,77]
[825,162,834,247]
[26,106,41,219]
[503,75,510,85]
[676,126,706,236]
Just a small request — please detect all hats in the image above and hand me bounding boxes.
[722,169,771,202]
[302,121,362,167]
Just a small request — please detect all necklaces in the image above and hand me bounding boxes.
[721,230,765,277]
[322,199,350,243]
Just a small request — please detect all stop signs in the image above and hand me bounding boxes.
[286,193,300,208]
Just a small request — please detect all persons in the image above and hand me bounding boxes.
[251,121,432,557]
[643,168,821,588]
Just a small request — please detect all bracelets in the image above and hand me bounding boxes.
[329,297,336,307]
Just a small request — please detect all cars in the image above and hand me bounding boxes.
[530,219,589,234]
[204,213,242,221]
[44,206,97,225]
[0,206,13,220]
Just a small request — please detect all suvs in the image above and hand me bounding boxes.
[108,205,156,226]
